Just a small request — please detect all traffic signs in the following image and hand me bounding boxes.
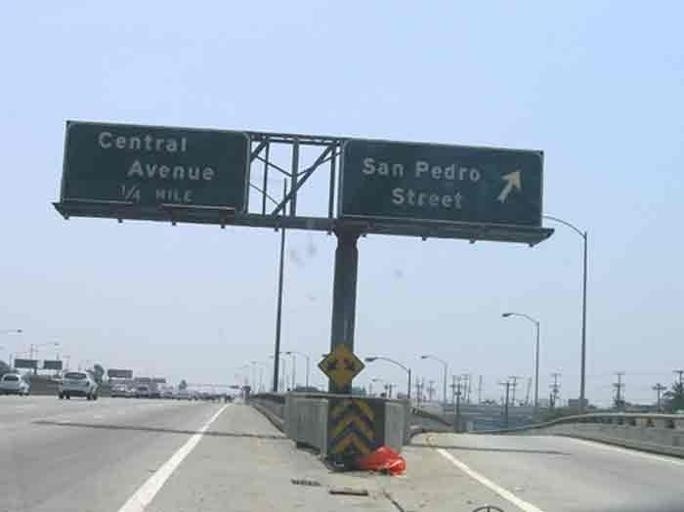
[342,138,543,227]
[63,121,248,211]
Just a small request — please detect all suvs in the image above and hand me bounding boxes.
[59,371,100,401]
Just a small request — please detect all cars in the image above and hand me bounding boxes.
[0,373,31,396]
[110,383,243,401]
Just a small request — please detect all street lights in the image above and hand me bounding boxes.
[365,356,413,398]
[237,349,312,392]
[0,329,23,334]
[500,309,541,407]
[422,355,448,401]
[30,341,61,357]
